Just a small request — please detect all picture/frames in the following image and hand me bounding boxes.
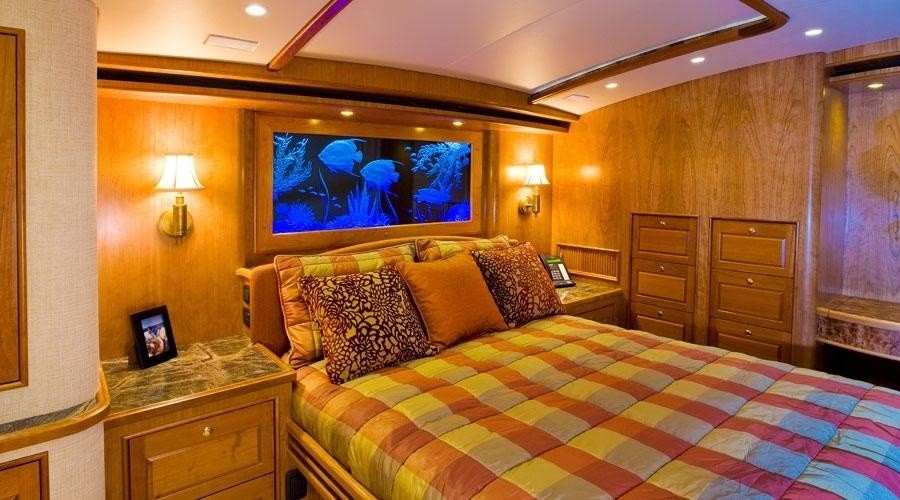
[131,305,178,371]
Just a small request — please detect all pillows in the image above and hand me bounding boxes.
[476,243,564,327]
[274,244,415,364]
[299,271,436,385]
[419,235,510,261]
[399,253,507,352]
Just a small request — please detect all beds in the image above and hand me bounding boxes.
[237,236,899,500]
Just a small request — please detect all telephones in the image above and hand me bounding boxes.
[537,253,576,289]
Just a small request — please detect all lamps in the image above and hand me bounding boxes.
[519,166,549,218]
[154,153,206,238]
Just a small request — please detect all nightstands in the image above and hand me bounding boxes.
[556,283,623,326]
[100,336,296,500]
